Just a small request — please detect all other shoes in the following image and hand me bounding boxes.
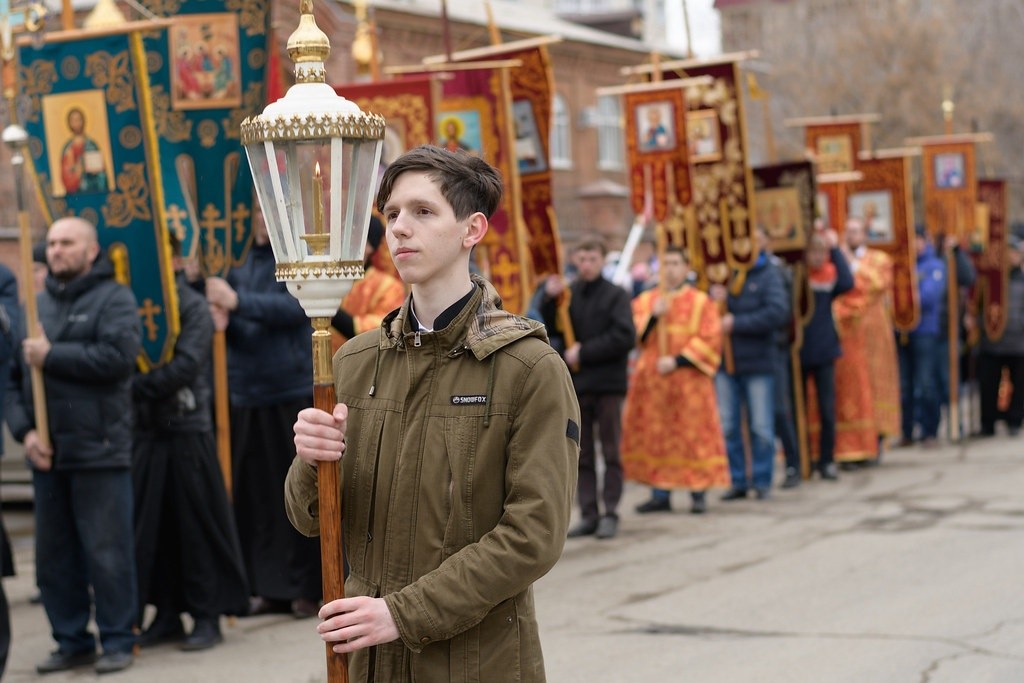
[95,652,131,672]
[293,599,315,619]
[36,648,95,672]
[756,487,768,499]
[597,515,617,537]
[567,519,597,538]
[181,612,222,652]
[257,598,291,612]
[781,471,802,488]
[721,489,748,501]
[692,498,705,512]
[138,610,182,646]
[636,498,672,512]
[820,460,835,480]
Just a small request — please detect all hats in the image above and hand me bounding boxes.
[32,238,46,263]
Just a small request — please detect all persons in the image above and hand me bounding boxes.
[0,262,23,680]
[285,144,581,683]
[123,230,250,652]
[9,218,141,675]
[529,215,1024,537]
[200,204,405,616]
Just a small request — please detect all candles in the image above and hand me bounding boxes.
[313,176,324,235]
